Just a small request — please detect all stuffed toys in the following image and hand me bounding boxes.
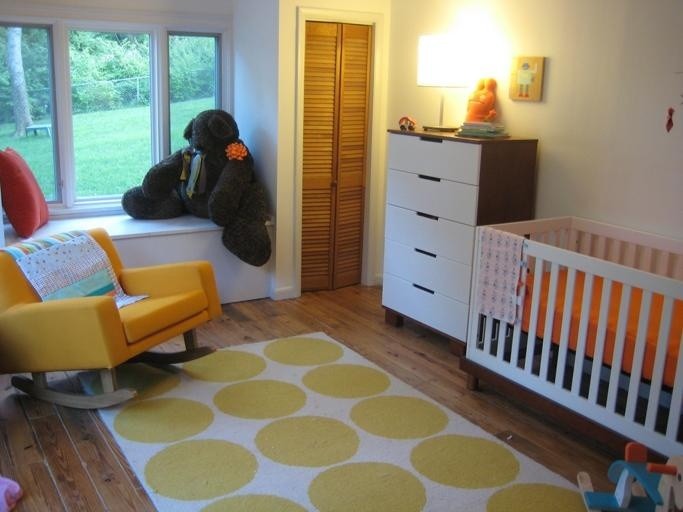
[123,109,272,266]
[463,77,497,124]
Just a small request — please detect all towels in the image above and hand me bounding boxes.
[478,227,525,326]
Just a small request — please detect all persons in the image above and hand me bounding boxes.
[517,62,534,97]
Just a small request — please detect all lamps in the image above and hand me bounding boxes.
[418,34,475,131]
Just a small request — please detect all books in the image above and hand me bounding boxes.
[454,122,512,139]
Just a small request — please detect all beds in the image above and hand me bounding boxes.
[459,214,681,464]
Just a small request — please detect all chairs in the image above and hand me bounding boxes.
[0,228,226,411]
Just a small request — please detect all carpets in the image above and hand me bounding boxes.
[77,329,586,511]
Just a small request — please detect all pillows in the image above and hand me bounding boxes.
[2,148,51,237]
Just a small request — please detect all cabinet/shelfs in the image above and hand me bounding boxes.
[381,126,538,357]
